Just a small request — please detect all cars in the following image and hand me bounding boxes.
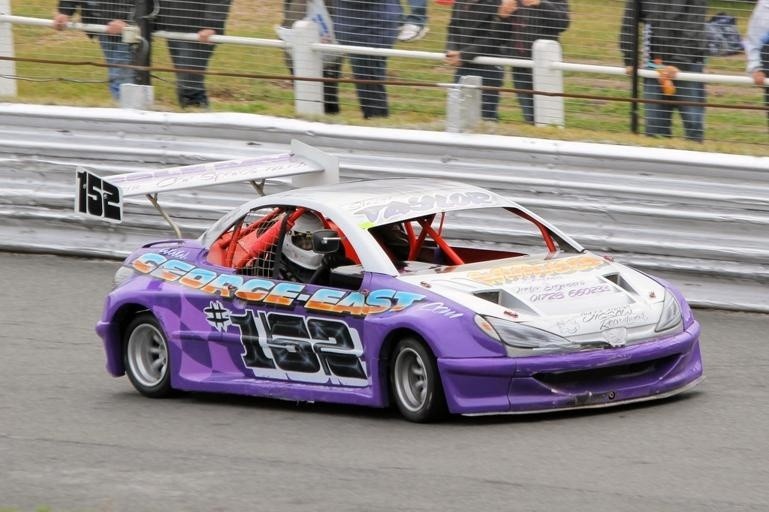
[72,138,707,425]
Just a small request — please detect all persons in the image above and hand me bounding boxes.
[740,0,769,133]
[144,0,232,113]
[445,0,512,123]
[53,1,155,105]
[617,0,710,142]
[487,0,573,126]
[280,1,432,124]
[272,207,363,290]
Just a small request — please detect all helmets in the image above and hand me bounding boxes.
[281,211,328,270]
